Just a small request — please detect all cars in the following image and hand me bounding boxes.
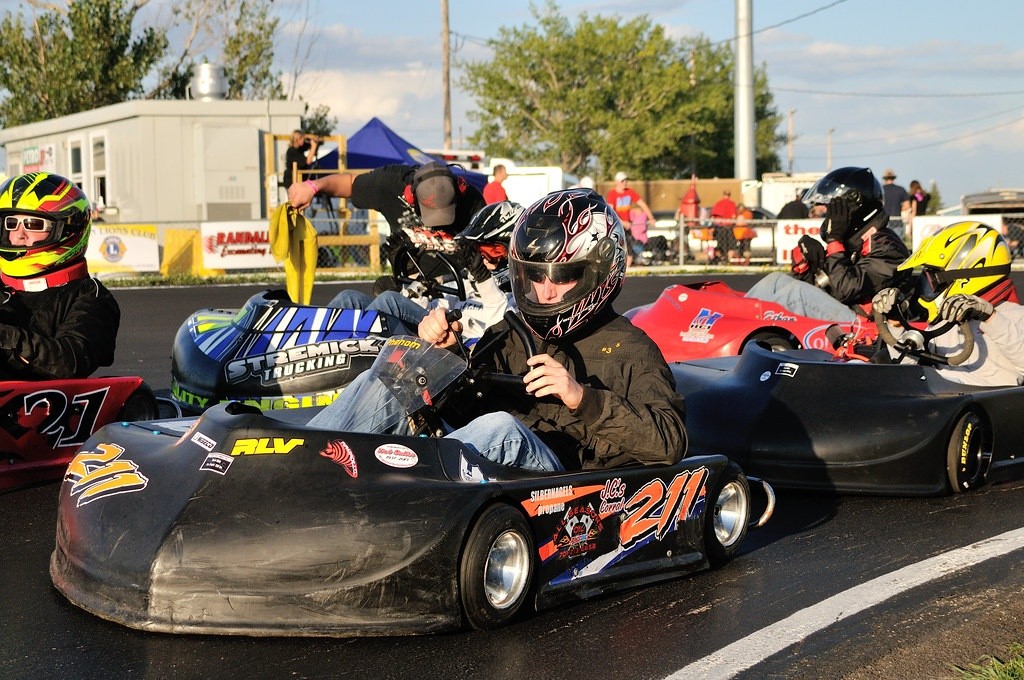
[647,206,778,265]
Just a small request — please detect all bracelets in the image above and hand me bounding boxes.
[305,179,318,194]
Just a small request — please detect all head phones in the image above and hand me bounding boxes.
[402,170,468,209]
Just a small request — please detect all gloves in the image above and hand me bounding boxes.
[454,234,492,283]
[872,287,910,322]
[819,196,851,243]
[938,294,996,323]
[798,235,827,276]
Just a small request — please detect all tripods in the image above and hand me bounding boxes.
[305,156,338,228]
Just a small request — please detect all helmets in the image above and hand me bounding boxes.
[0,172,93,278]
[508,188,628,341]
[453,201,527,297]
[897,220,1013,325]
[814,167,885,244]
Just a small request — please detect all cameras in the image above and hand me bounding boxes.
[304,138,324,146]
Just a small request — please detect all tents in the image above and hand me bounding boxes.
[307,117,489,195]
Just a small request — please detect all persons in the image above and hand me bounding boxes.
[288,164,488,296]
[908,179,932,216]
[327,200,526,352]
[879,167,911,242]
[482,164,510,205]
[307,187,689,478]
[284,129,318,202]
[794,166,916,326]
[605,171,810,268]
[0,171,122,381]
[871,221,1024,394]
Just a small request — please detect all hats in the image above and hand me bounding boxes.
[616,172,629,182]
[414,161,456,227]
[882,170,896,180]
[581,177,597,191]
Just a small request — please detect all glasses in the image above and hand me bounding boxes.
[923,267,945,293]
[5,215,54,232]
[524,261,591,285]
[477,241,507,264]
